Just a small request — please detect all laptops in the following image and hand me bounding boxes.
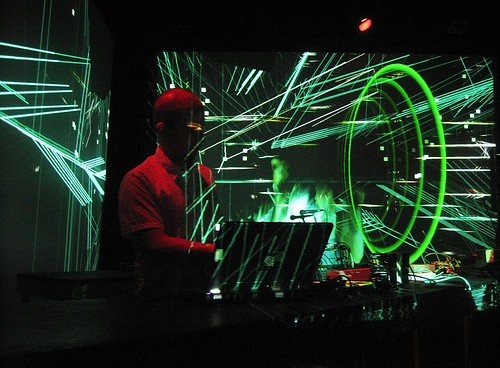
[147,221,334,307]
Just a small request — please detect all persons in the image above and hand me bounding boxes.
[116,88,219,276]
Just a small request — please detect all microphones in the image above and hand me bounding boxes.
[288,214,312,220]
[299,207,322,215]
